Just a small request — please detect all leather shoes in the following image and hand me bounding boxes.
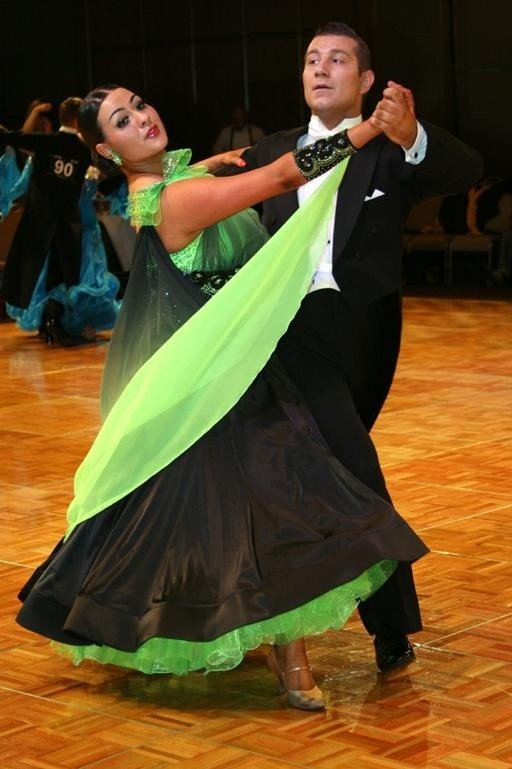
[374,633,414,672]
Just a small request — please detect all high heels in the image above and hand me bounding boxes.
[266,645,327,710]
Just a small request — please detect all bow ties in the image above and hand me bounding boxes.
[308,119,343,141]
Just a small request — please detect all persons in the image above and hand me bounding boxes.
[0,96,118,348]
[16,79,431,709]
[0,82,128,341]
[125,15,480,682]
[213,104,267,216]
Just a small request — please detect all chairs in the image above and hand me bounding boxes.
[408,193,456,289]
[450,192,499,287]
